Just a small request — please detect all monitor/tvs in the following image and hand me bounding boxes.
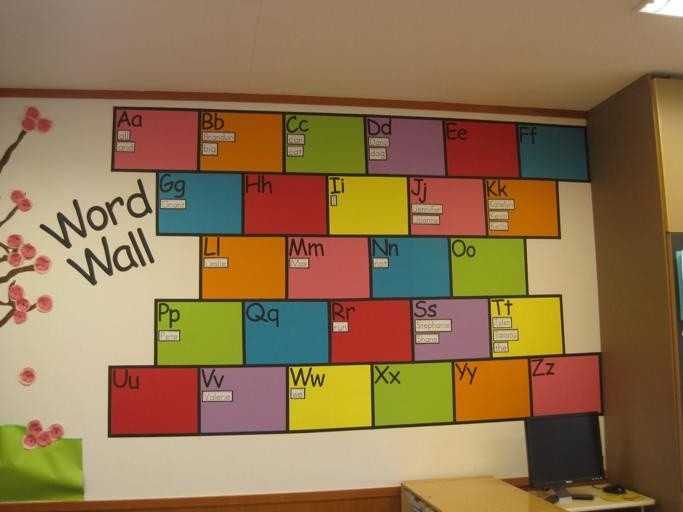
[524,411,607,503]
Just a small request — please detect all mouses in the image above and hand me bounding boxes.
[603,485,626,493]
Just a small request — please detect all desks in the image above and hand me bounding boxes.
[397,475,565,512]
[521,477,659,512]
